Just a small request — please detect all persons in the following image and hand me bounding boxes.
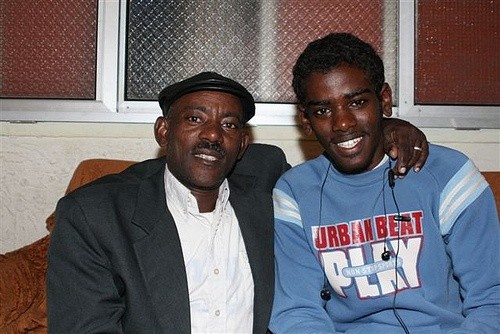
[42,70,429,333]
[269,30,500,334]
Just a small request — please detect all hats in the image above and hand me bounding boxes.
[158,72,255,124]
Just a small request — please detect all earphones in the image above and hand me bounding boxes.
[382,251,390,261]
[320,290,332,301]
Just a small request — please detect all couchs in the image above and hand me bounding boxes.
[0,159,500,334]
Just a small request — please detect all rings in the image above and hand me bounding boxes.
[414,146,424,152]
